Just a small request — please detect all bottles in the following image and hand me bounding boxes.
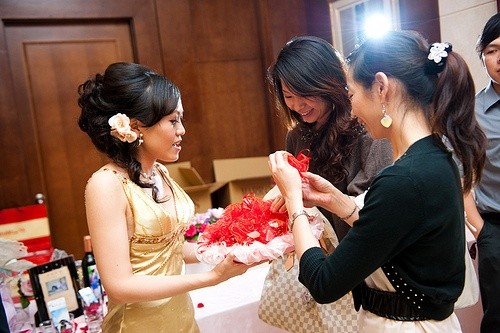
[80,235,104,308]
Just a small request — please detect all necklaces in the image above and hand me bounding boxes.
[140,165,156,180]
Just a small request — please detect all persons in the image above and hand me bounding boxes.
[267,30,489,333]
[264,35,395,245]
[441,14,500,333]
[77,60,270,333]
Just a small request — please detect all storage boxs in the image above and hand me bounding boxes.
[164,156,275,213]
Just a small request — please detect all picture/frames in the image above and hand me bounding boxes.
[29,256,84,324]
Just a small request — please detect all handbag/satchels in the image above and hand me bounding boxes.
[259,219,360,333]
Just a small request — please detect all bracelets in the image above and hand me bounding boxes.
[340,204,358,220]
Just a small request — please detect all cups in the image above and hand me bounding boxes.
[79,293,104,333]
[9,312,76,333]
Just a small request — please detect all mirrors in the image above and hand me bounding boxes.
[329,0,402,61]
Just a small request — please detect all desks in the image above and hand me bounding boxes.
[12,243,293,333]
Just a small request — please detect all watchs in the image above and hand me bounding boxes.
[288,210,309,234]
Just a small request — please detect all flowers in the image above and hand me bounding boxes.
[107,114,138,143]
[184,204,226,246]
